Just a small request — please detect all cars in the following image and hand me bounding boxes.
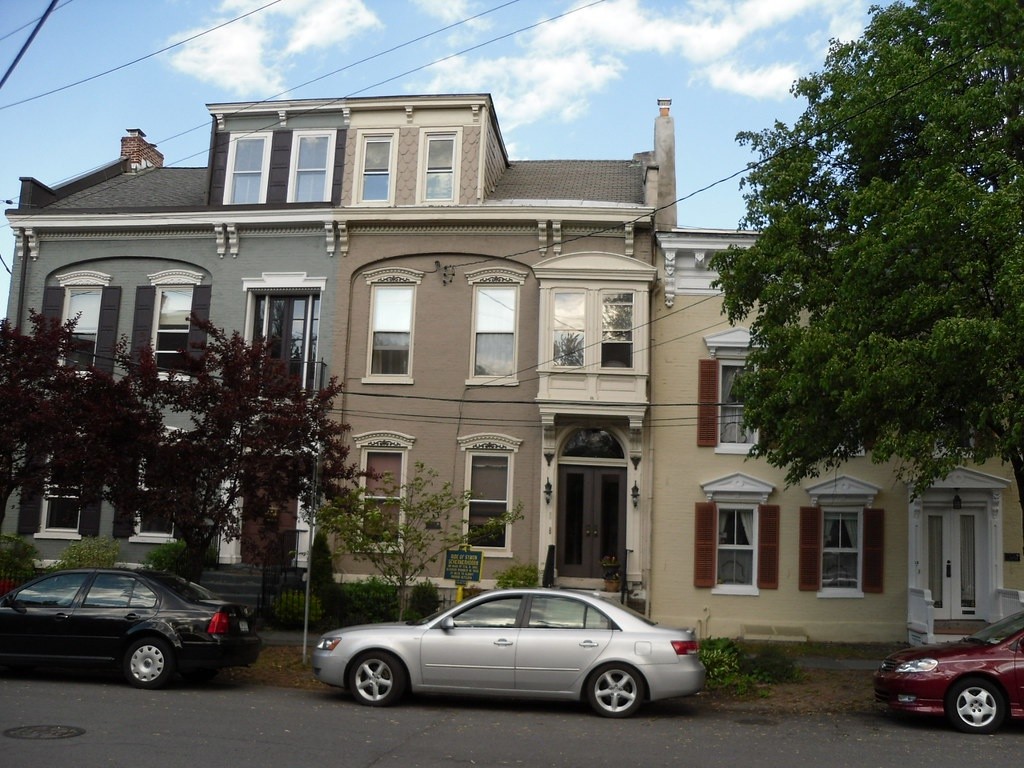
[874,611,1024,735]
[312,588,706,719]
[0,567,261,690]
